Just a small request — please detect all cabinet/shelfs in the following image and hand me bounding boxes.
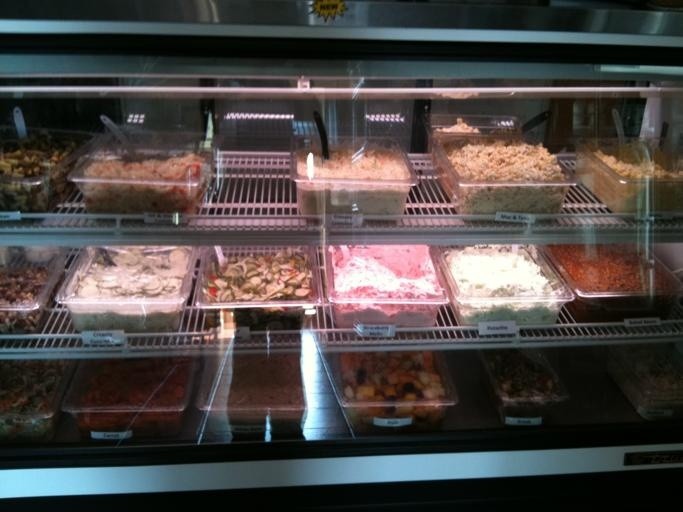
[0,20,681,499]
